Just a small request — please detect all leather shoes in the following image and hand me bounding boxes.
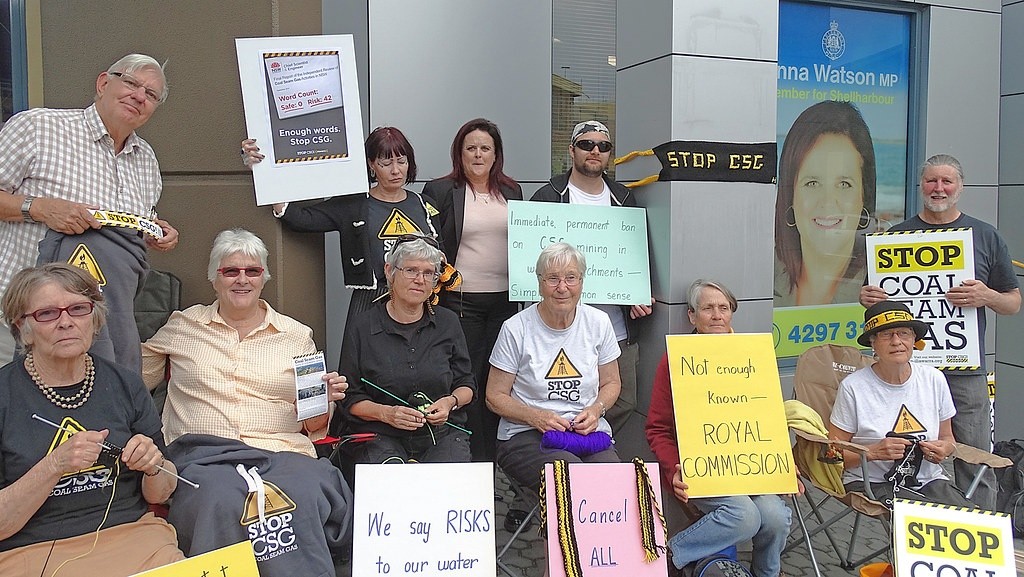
[504,509,532,532]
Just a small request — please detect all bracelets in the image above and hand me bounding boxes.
[21,195,38,223]
[596,401,605,417]
[143,449,165,476]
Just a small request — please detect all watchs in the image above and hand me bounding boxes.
[450,394,458,411]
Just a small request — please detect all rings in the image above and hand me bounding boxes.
[964,299,966,304]
[929,452,934,456]
[241,148,244,154]
[340,390,346,393]
[928,456,932,461]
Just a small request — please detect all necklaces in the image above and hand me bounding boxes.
[480,195,489,203]
[26,349,94,407]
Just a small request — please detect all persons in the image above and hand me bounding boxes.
[859,154,1021,512]
[646,278,806,577]
[335,240,478,466]
[0,53,180,370]
[484,242,622,493]
[138,226,354,577]
[424,118,525,500]
[829,301,978,510]
[773,99,886,310]
[240,126,447,339]
[0,262,188,577]
[529,120,656,436]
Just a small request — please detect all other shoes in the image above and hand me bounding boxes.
[667,548,683,577]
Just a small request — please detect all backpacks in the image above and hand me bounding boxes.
[992,438,1024,538]
[693,546,754,577]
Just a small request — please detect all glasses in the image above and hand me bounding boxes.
[573,139,615,153]
[538,273,581,286]
[215,266,265,278]
[874,329,915,340]
[394,266,439,282]
[20,301,93,322]
[111,71,162,105]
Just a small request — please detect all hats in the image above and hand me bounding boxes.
[857,301,928,347]
[571,120,610,145]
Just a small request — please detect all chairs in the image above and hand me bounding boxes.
[315,344,1013,577]
[132,269,182,412]
